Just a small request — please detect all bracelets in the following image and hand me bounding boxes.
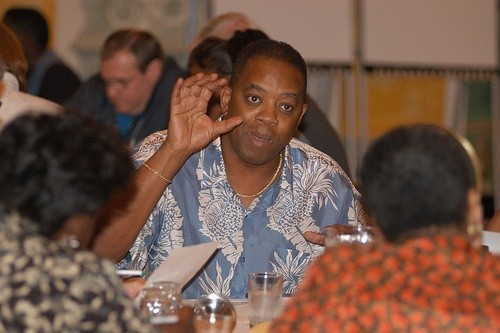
[142,162,172,184]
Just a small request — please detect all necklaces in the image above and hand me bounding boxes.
[235,152,282,197]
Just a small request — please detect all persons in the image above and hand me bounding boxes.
[90,39,372,299]
[1,109,156,333]
[267,123,500,333]
[1,5,351,181]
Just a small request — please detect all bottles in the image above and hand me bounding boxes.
[140,279,237,333]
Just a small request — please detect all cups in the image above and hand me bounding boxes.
[248,271,283,329]
[322,223,376,252]
